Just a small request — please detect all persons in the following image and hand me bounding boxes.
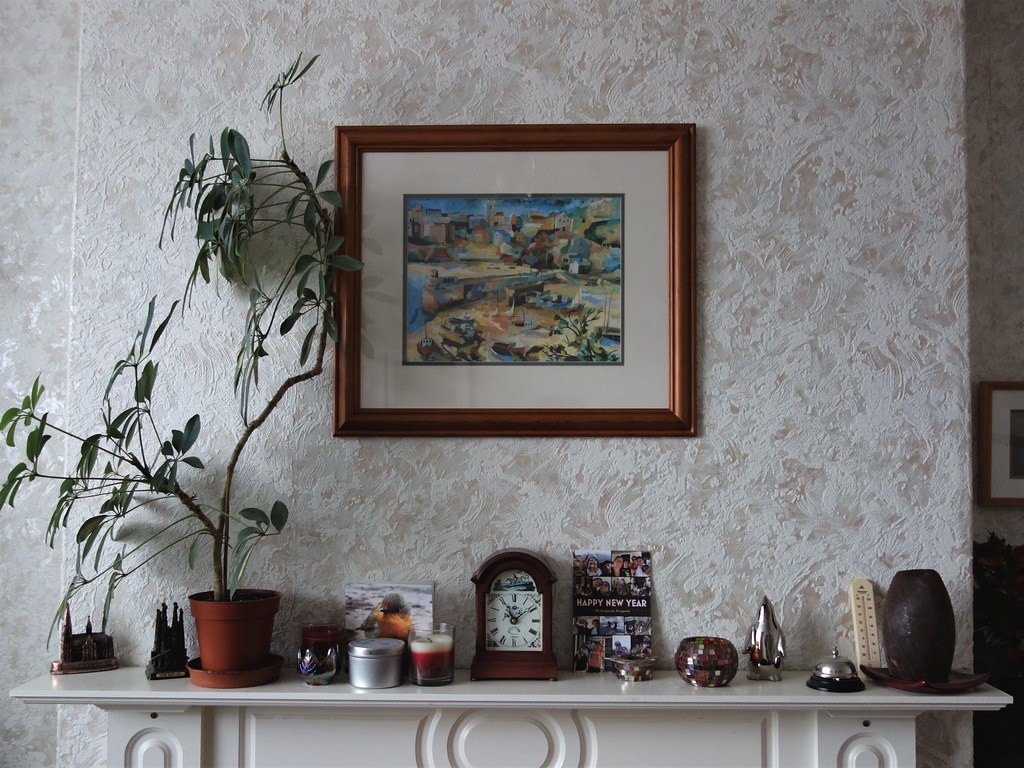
[573,550,652,672]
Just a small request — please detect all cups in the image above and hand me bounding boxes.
[299,618,346,677]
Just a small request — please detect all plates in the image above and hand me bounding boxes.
[859,665,989,692]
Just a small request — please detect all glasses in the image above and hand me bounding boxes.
[601,583,607,587]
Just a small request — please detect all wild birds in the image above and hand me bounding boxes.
[354,593,415,645]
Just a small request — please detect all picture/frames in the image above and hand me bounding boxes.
[332,123,698,438]
[978,380,1023,508]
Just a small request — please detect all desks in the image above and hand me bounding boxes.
[9,666,1014,768]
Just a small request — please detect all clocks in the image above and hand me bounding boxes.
[471,548,559,682]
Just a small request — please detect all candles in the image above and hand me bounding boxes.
[410,634,454,680]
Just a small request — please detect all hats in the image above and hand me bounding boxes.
[600,560,613,568]
[631,556,637,560]
[573,558,580,567]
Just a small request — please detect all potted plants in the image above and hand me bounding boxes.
[0,51,365,689]
